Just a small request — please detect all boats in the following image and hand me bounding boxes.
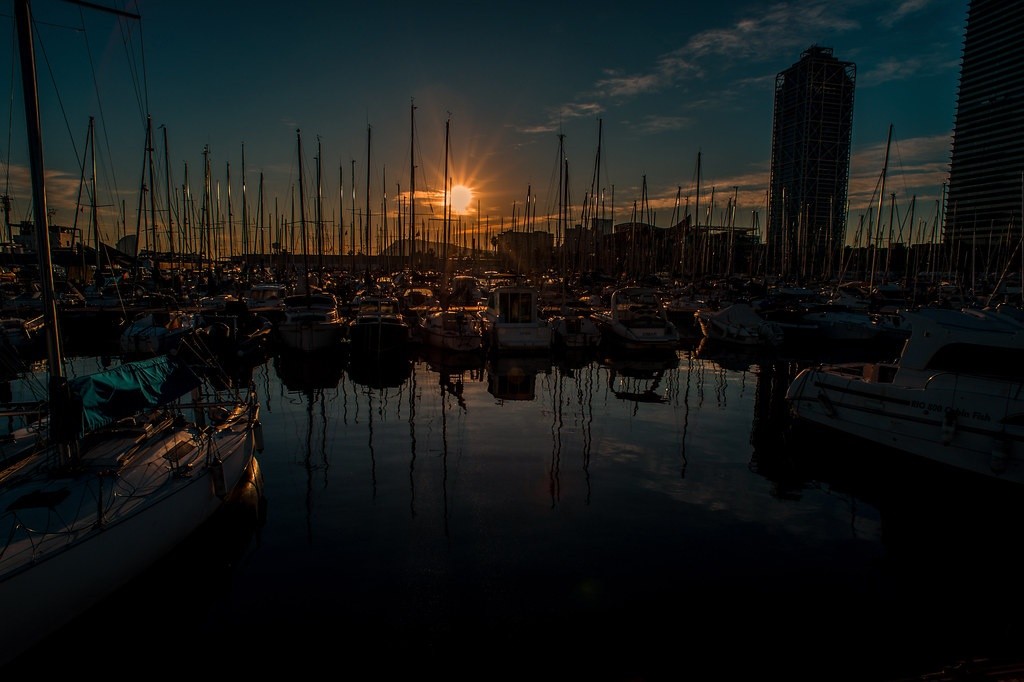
[787,224,1024,492]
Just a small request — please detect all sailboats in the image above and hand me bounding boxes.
[0,0,264,650]
[1,1,1024,361]
